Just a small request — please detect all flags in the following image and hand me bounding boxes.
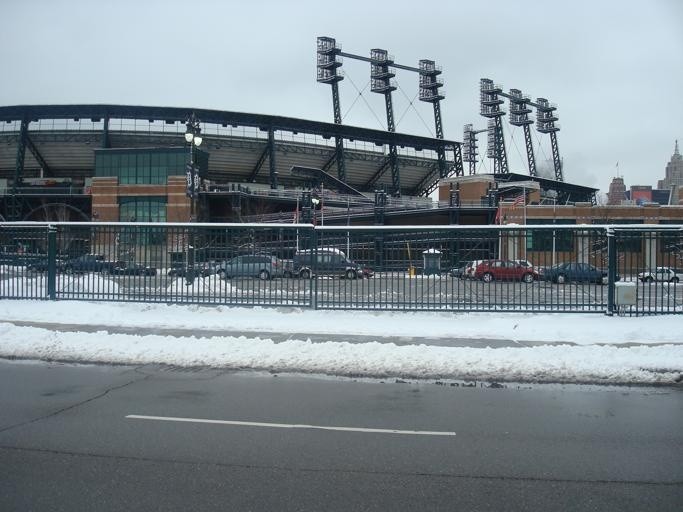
[493,197,502,225]
[510,187,525,211]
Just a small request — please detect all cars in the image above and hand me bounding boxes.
[448,257,622,286]
[638,266,683,283]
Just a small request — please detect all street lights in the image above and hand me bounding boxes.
[183,111,203,287]
[311,191,321,225]
